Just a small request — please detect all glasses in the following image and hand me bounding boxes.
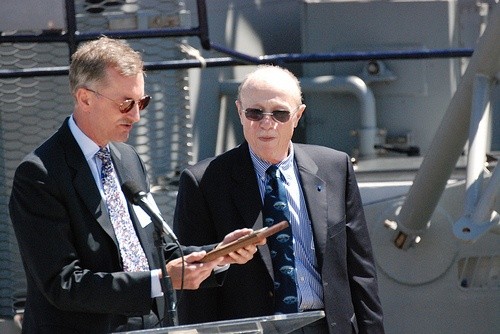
[239,105,297,123]
[86,88,151,113]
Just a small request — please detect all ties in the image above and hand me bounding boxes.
[97,148,149,272]
[262,165,298,315]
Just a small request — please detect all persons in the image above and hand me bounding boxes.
[172,66,386,334]
[9,39,267,334]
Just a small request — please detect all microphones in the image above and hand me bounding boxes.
[121,181,178,245]
